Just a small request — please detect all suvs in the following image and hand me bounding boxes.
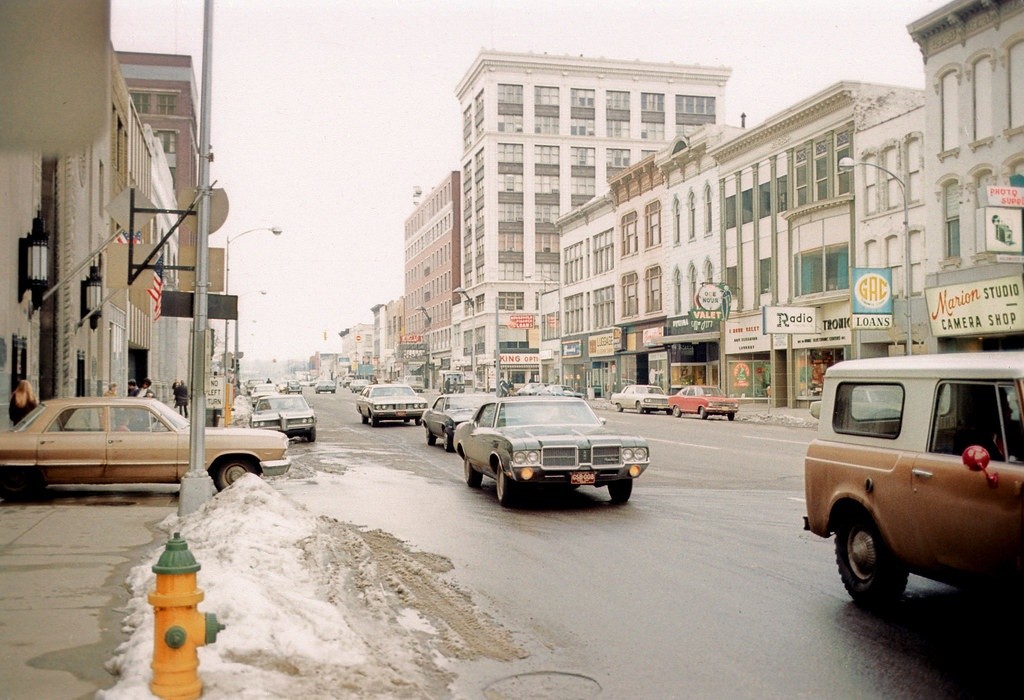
[804,343,1023,633]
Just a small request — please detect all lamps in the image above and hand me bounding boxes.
[80,266,103,332]
[18,217,49,310]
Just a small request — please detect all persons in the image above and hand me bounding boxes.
[445,378,450,393]
[423,377,429,388]
[173,378,188,418]
[952,388,1013,457]
[266,378,271,383]
[508,378,514,397]
[451,378,457,393]
[103,382,117,397]
[127,379,137,397]
[130,379,155,398]
[115,411,131,432]
[374,377,377,384]
[8,379,37,426]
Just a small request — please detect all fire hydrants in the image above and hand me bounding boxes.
[147,530,228,699]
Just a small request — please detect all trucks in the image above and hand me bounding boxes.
[439,370,465,394]
[404,375,425,393]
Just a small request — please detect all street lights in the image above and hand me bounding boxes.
[222,291,267,384]
[838,156,922,359]
[224,225,283,383]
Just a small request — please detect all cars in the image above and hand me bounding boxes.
[668,385,739,421]
[0,397,293,495]
[516,382,586,400]
[245,378,318,443]
[421,394,501,452]
[453,395,651,505]
[356,384,428,426]
[316,380,336,394]
[589,377,636,396]
[339,376,374,393]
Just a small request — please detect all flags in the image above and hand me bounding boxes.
[147,253,164,320]
[114,229,141,245]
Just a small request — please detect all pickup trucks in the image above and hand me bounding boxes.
[611,384,673,414]
